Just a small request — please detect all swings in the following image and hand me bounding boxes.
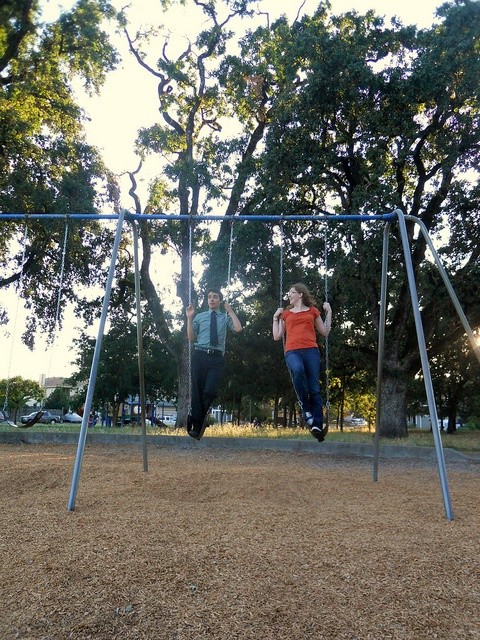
[1,221,69,428]
[186,220,234,440]
[280,220,330,439]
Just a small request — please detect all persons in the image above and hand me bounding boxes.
[272,284,333,442]
[185,289,243,438]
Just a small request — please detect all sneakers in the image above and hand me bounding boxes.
[305,412,314,426]
[312,426,324,442]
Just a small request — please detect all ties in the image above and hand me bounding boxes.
[210,311,218,346]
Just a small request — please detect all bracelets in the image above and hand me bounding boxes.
[273,320,278,322]
[273,316,278,318]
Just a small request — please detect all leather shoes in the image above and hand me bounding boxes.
[189,430,198,437]
[187,415,192,433]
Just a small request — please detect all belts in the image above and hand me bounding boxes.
[195,346,222,356]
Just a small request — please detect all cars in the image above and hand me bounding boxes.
[160,416,176,426]
[60,412,82,423]
[20,411,60,425]
[0,411,4,419]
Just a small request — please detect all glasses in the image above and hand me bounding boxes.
[288,291,298,294]
